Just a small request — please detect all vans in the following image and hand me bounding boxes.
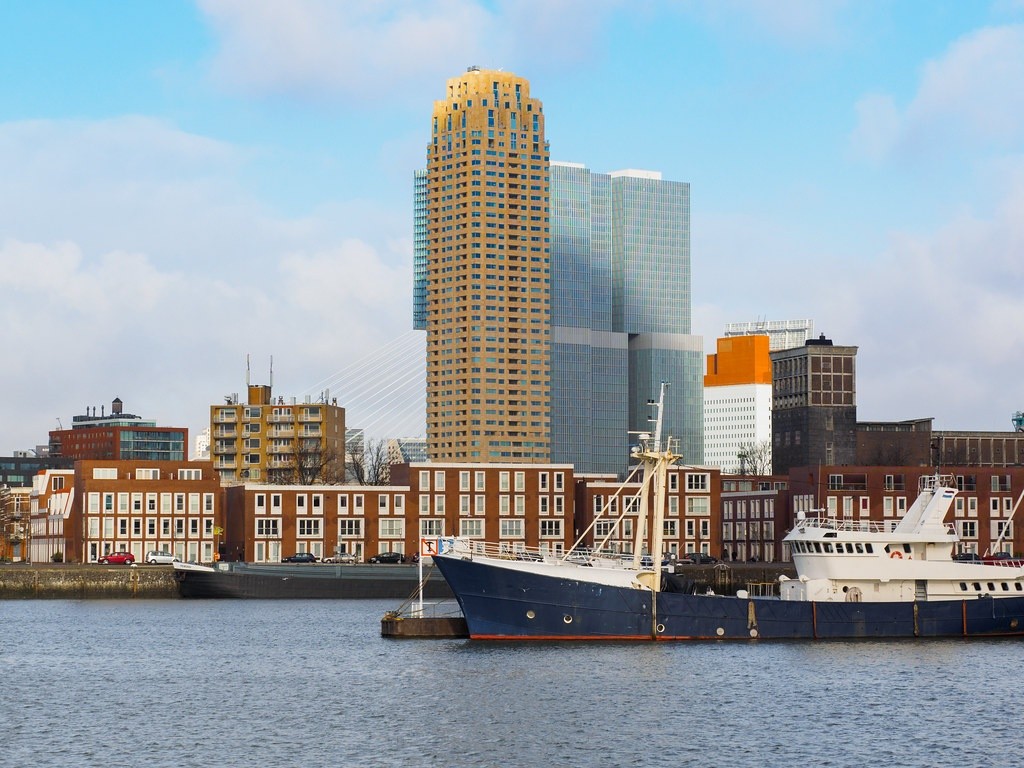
[145,551,178,564]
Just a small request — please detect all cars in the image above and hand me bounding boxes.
[97,551,135,565]
[323,553,357,563]
[368,551,402,565]
[279,552,315,563]
[688,552,716,563]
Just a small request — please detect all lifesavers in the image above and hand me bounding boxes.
[890,550,903,559]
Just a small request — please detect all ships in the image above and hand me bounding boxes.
[433,385,1024,639]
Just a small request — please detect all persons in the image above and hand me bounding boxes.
[977,593,993,599]
[951,553,958,560]
[986,552,991,557]
[821,521,830,528]
[909,555,912,559]
